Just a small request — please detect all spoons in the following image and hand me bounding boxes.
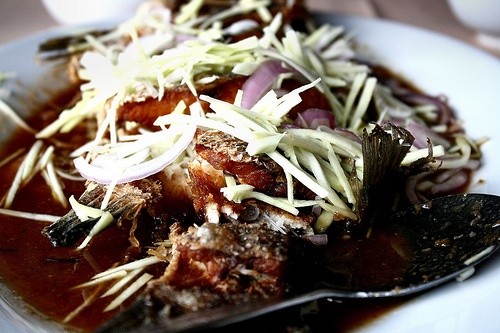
[124,193,499,333]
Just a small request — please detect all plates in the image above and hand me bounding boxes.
[0,7,500,331]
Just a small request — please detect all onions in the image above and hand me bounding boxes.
[74,56,481,203]
